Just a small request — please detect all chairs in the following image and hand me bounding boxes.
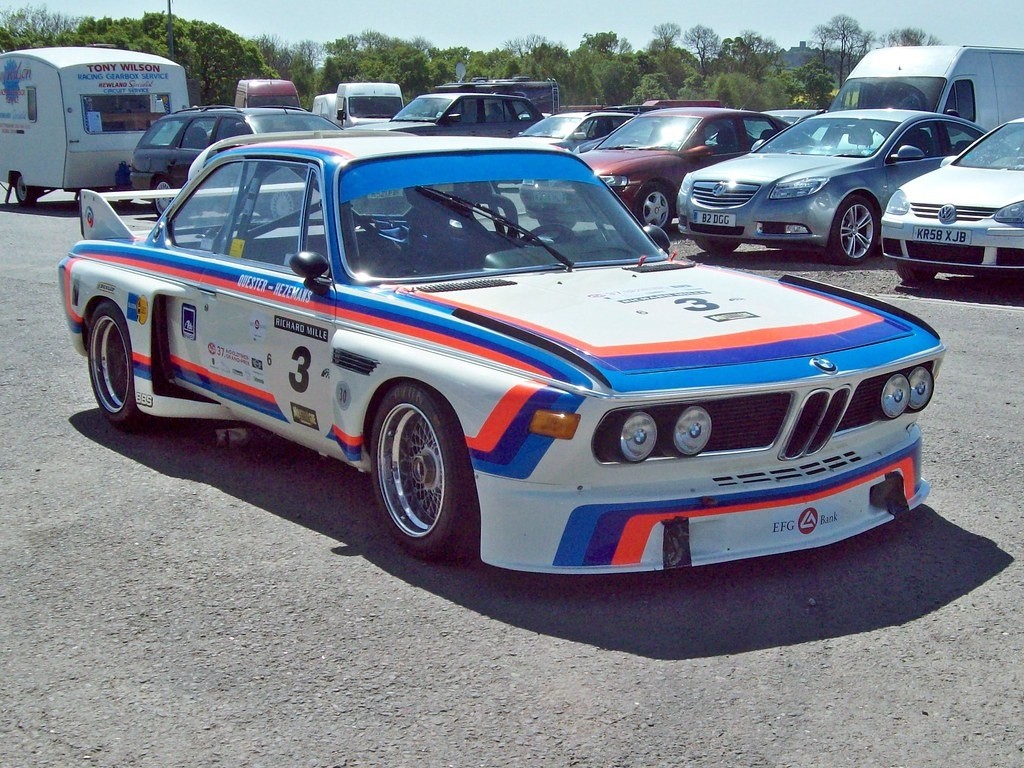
[850,124,873,152]
[714,127,735,154]
[896,130,931,161]
[186,127,208,149]
[409,193,519,275]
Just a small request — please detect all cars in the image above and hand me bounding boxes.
[672,107,1017,268]
[522,105,821,235]
[310,78,717,187]
[702,108,825,149]
[879,116,1024,290]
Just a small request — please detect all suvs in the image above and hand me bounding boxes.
[345,90,544,144]
[128,105,343,220]
[504,107,655,154]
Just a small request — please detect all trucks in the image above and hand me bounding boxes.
[0,46,191,206]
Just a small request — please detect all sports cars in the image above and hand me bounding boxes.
[54,129,948,580]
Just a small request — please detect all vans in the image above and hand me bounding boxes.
[232,77,302,110]
[810,45,1024,152]
